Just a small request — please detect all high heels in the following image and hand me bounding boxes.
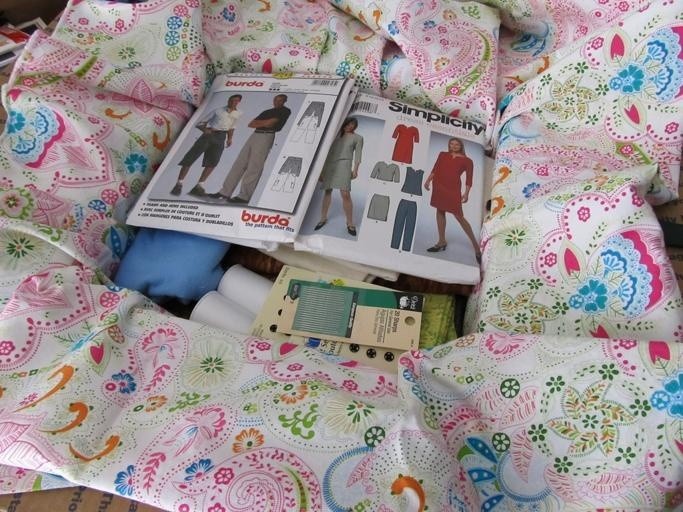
[426,244,447,252]
[476,251,482,264]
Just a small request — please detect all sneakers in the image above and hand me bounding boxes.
[190,184,207,195]
[171,182,182,196]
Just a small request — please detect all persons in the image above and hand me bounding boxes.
[424,138,482,264]
[169,94,244,198]
[204,94,292,205]
[312,117,363,236]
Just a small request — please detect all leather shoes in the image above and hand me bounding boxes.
[208,192,231,201]
[314,218,327,231]
[227,196,249,204]
[346,222,356,236]
